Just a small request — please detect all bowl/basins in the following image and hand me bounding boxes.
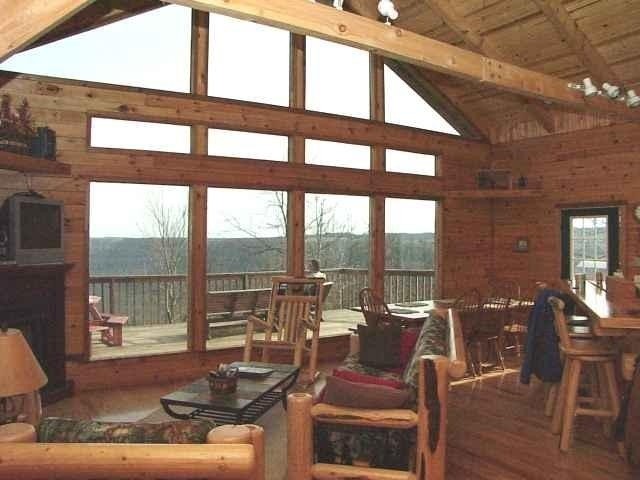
[207,375,236,394]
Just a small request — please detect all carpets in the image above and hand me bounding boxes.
[134,388,290,479]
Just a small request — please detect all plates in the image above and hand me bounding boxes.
[231,365,275,377]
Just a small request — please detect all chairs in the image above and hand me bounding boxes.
[534,280,593,325]
[342,273,537,380]
[1,416,267,480]
[243,276,328,382]
[541,284,610,417]
[547,295,623,454]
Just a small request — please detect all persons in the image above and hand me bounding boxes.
[303,258,327,277]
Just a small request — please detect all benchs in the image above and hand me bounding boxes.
[105,314,129,346]
[286,307,466,479]
[206,279,335,338]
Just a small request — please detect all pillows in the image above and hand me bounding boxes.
[316,368,405,405]
[321,375,407,409]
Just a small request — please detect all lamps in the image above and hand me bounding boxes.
[0,321,48,420]
[376,0,400,25]
[566,76,640,109]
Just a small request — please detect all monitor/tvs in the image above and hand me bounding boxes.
[0,195,65,266]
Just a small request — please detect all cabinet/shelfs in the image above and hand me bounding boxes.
[1,264,74,407]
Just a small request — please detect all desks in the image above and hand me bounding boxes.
[555,273,639,469]
[88,296,114,344]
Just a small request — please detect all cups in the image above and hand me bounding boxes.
[596,272,602,281]
[575,274,585,289]
